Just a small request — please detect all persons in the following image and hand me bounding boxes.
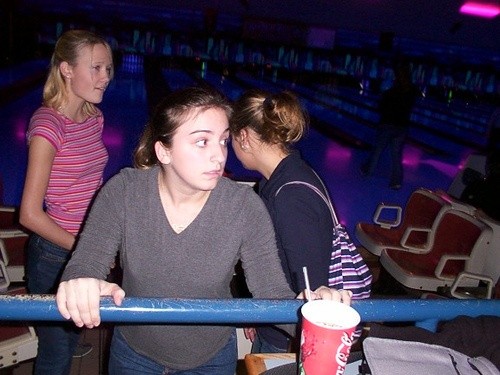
[361,60,421,189]
[230,86,335,354]
[56,84,353,375]
[18,29,114,375]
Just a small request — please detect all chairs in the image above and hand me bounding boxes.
[356,190,500,299]
[0,207,39,369]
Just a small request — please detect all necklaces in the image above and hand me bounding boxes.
[173,222,184,234]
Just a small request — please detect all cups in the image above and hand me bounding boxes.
[299,300,361,375]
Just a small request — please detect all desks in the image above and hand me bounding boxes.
[245,352,300,375]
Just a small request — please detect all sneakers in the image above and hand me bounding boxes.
[71,344,93,358]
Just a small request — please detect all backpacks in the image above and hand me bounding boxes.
[274,168,373,300]
